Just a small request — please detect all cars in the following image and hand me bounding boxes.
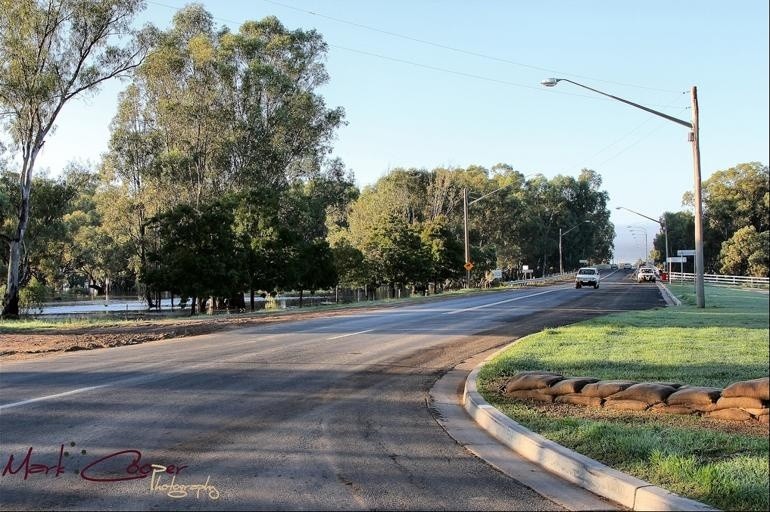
[611,264,617,269]
[637,267,656,283]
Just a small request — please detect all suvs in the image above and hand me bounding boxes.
[575,267,601,288]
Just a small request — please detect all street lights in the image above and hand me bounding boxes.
[542,77,705,309]
[616,206,669,272]
[464,172,541,289]
[559,220,590,274]
